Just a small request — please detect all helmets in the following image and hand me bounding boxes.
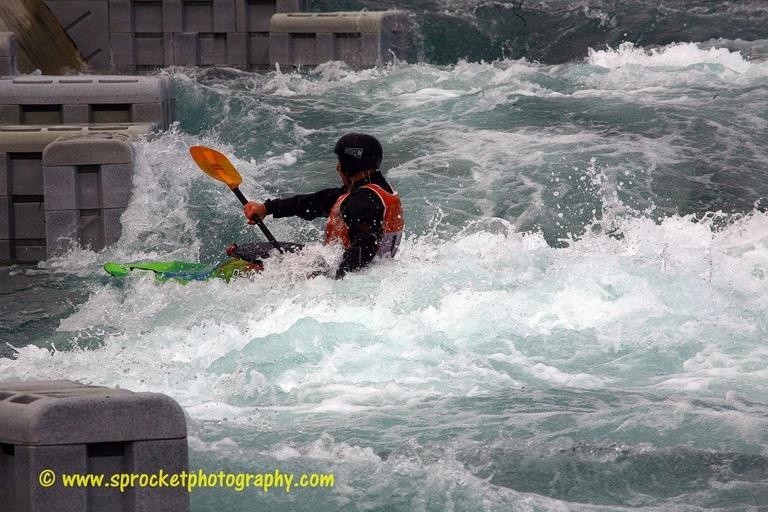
[334,133,382,178]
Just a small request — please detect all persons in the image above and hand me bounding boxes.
[242,133,404,280]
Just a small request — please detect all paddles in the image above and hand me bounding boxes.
[190,145,282,252]
